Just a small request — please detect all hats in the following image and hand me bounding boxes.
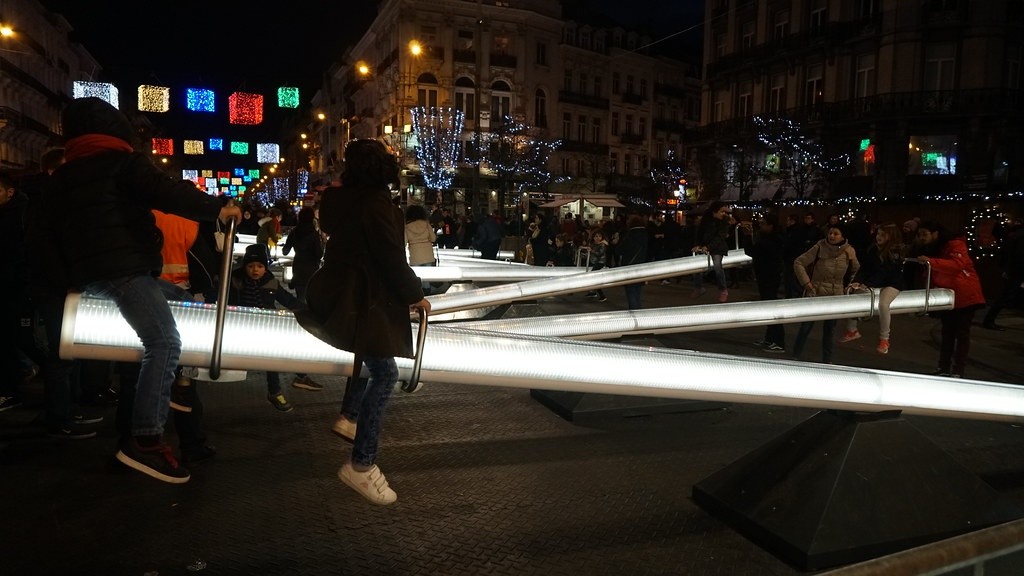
[299,207,315,225]
[270,207,282,216]
[243,243,267,269]
[904,217,920,232]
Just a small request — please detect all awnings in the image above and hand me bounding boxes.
[586,198,625,207]
[538,199,577,207]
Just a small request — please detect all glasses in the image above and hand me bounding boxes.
[917,231,931,238]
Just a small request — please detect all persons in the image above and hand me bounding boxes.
[0,148,217,474]
[839,222,908,354]
[917,219,986,377]
[23,98,242,483]
[217,170,342,411]
[742,213,785,354]
[430,205,663,310]
[640,200,1024,332]
[404,204,437,296]
[794,225,861,361]
[291,139,431,505]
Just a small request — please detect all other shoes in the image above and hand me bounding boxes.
[984,322,1007,330]
[0,392,23,414]
[938,370,963,379]
[80,382,122,408]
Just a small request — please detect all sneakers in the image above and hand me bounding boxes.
[292,375,323,391]
[839,330,861,343]
[182,440,217,467]
[719,289,728,303]
[104,451,129,474]
[689,287,706,299]
[64,408,104,425]
[116,437,190,485]
[331,412,357,445]
[660,280,671,285]
[752,339,785,354]
[165,385,192,415]
[47,423,96,440]
[876,339,890,354]
[268,390,294,413]
[338,457,396,504]
[585,292,597,298]
[598,297,607,302]
